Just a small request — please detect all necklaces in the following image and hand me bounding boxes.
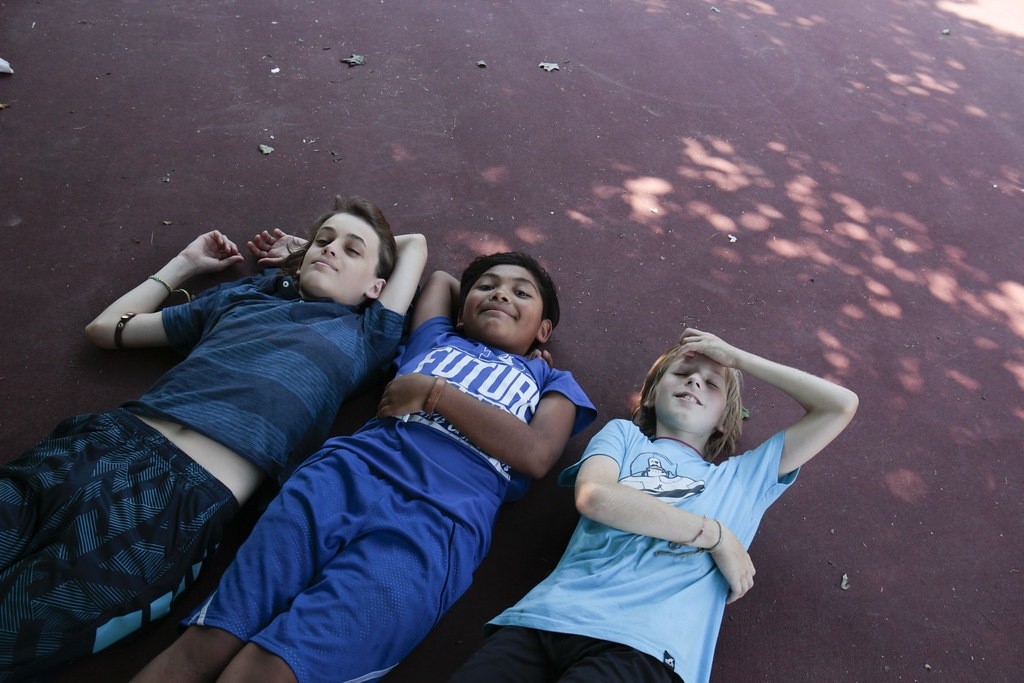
[652,435,703,457]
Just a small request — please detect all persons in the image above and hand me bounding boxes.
[2,192,428,682]
[127,249,598,683]
[449,329,859,683]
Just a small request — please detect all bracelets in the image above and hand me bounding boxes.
[146,276,173,294]
[423,369,446,413]
[115,311,135,350]
[699,516,723,553]
[669,513,707,551]
